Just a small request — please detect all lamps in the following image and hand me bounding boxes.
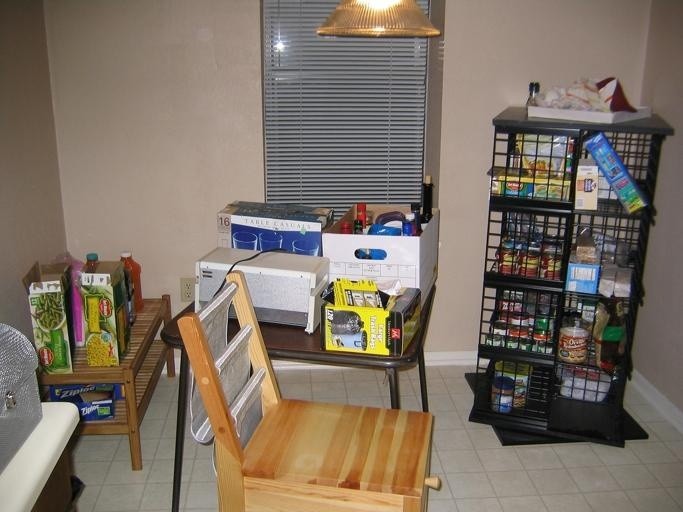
[316,0,441,38]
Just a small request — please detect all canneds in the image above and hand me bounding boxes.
[500,233,564,281]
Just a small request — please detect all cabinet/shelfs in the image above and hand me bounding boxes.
[464,105,675,448]
[35,294,175,471]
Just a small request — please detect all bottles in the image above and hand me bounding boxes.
[119,252,144,311]
[526,82,540,109]
[402,203,423,236]
[342,203,372,234]
[420,183,433,231]
[85,253,98,275]
[574,302,582,327]
[490,376,514,414]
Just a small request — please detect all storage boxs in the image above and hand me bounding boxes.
[49,384,116,423]
[0,402,83,512]
[22,261,76,375]
[215,199,334,256]
[319,287,422,361]
[77,261,132,368]
[322,204,440,312]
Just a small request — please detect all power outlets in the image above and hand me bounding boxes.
[179,277,195,303]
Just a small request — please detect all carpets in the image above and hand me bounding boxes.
[464,373,650,446]
